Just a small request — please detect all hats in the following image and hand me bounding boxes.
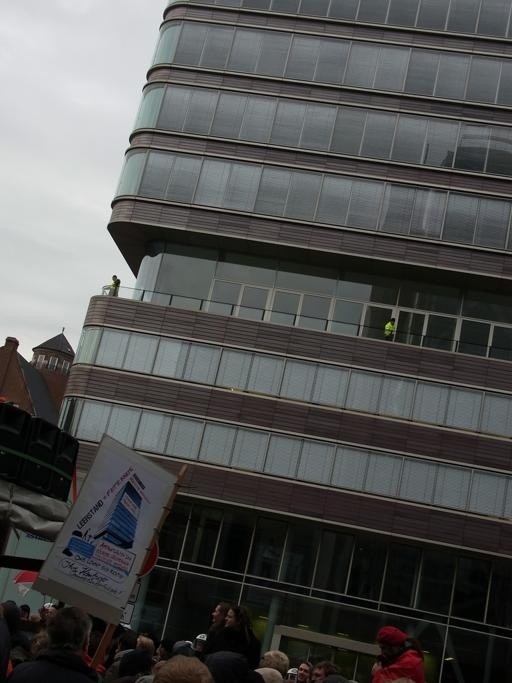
[195,633,208,643]
[375,626,409,648]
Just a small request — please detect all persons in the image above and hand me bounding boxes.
[109,274,121,296]
[384,317,396,340]
[371,625,426,682]
